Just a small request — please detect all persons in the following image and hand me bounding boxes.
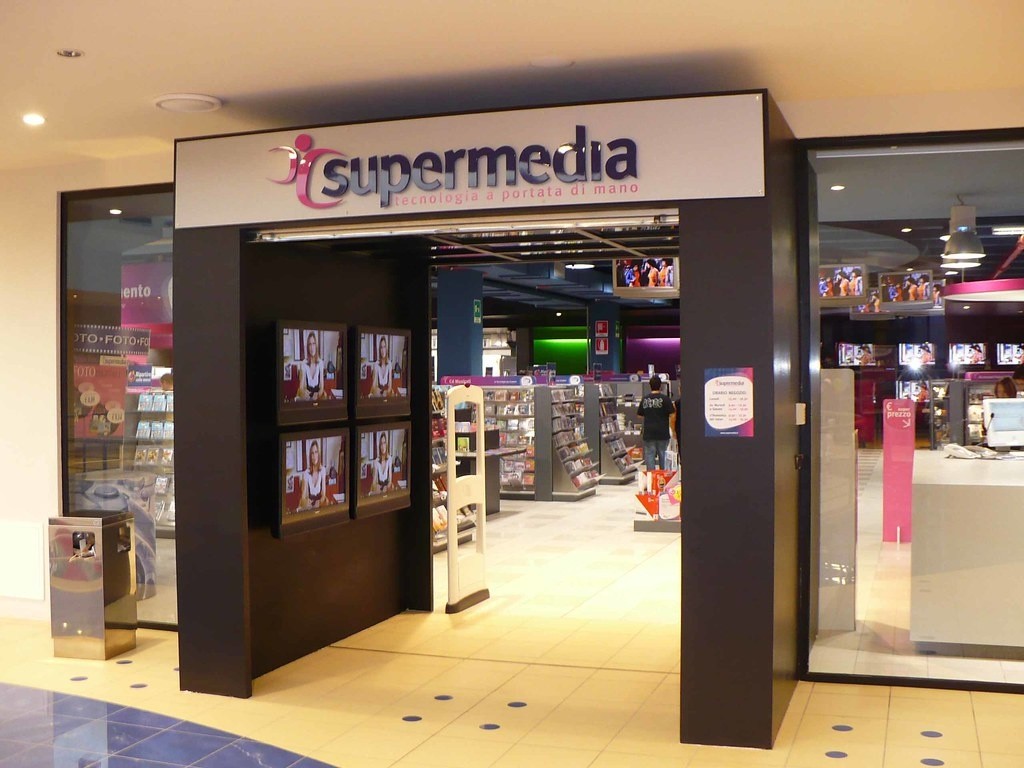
[616,258,674,287]
[371,433,394,494]
[299,332,324,400]
[913,382,928,402]
[993,376,1016,398]
[673,382,681,464]
[1012,365,1024,392]
[298,440,326,509]
[636,375,676,470]
[372,336,392,396]
[819,267,1024,365]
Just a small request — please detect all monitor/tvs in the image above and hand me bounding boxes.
[948,343,987,366]
[836,342,876,367]
[996,343,1024,366]
[612,258,680,299]
[276,321,411,537]
[897,343,937,367]
[983,399,1024,452]
[818,264,945,321]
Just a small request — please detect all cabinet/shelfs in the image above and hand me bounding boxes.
[121,388,176,539]
[926,377,998,449]
[433,380,681,557]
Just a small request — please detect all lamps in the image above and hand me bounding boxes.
[940,258,981,268]
[942,195,987,260]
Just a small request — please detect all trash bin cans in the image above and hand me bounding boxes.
[76,467,159,602]
[46,509,139,662]
